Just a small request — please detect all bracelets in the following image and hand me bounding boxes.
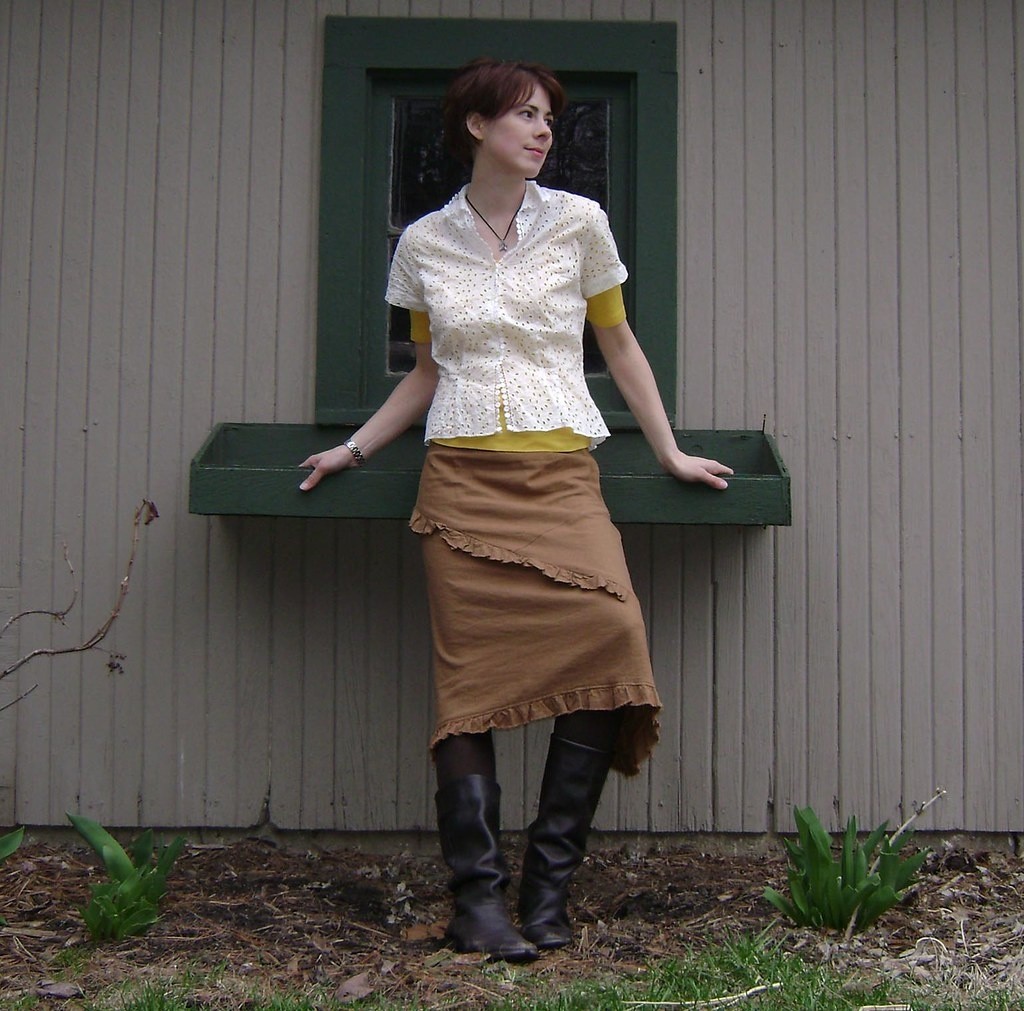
[343,438,367,468]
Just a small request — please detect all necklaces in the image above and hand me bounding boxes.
[465,194,524,251]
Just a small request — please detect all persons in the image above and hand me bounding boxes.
[297,57,734,960]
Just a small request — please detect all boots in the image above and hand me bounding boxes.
[520,733,615,948]
[434,773,540,959]
